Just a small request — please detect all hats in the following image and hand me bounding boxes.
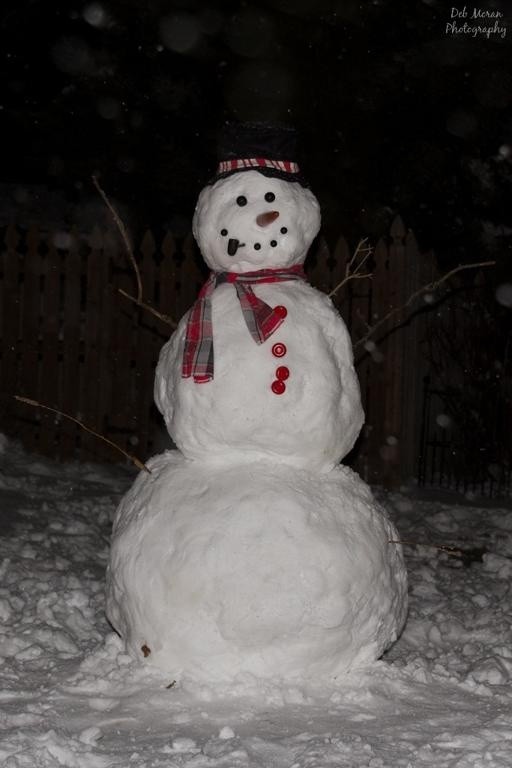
[212,123,309,182]
